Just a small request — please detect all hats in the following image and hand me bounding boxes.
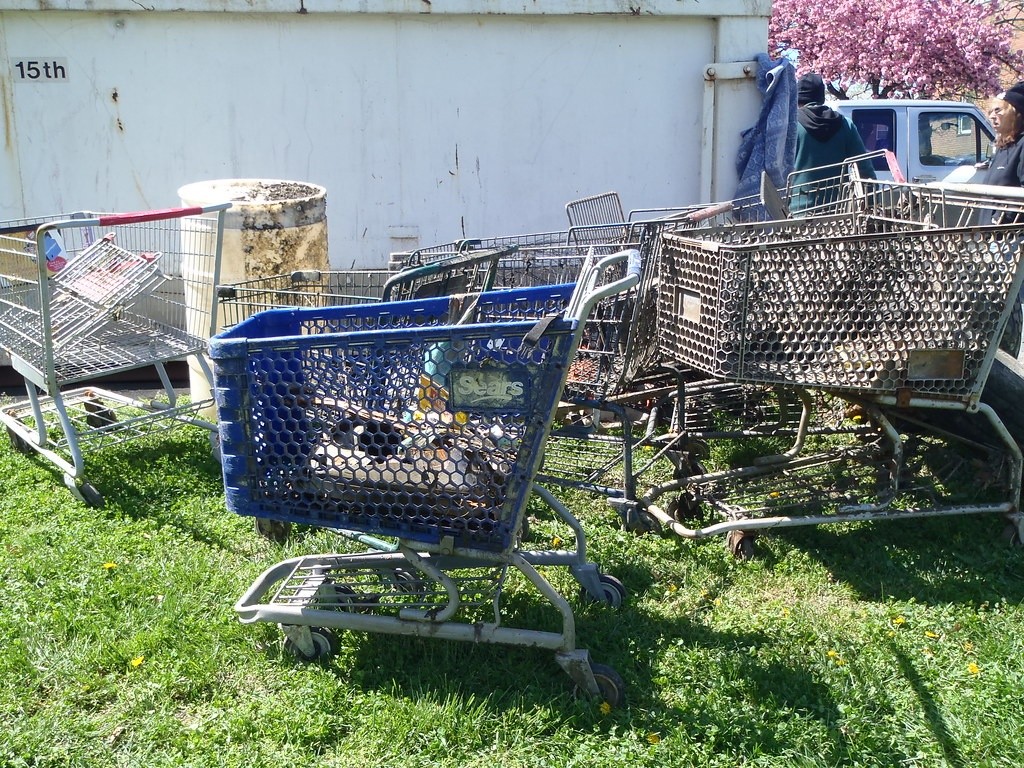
[796,72,825,104]
[995,81,1024,115]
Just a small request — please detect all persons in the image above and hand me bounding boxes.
[976,81,1024,239]
[790,73,878,217]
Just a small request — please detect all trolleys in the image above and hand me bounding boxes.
[0,202,236,508]
[208,148,1024,711]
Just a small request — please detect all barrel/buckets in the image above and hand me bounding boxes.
[178,178,332,429]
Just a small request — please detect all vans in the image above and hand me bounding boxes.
[828,100,998,189]
